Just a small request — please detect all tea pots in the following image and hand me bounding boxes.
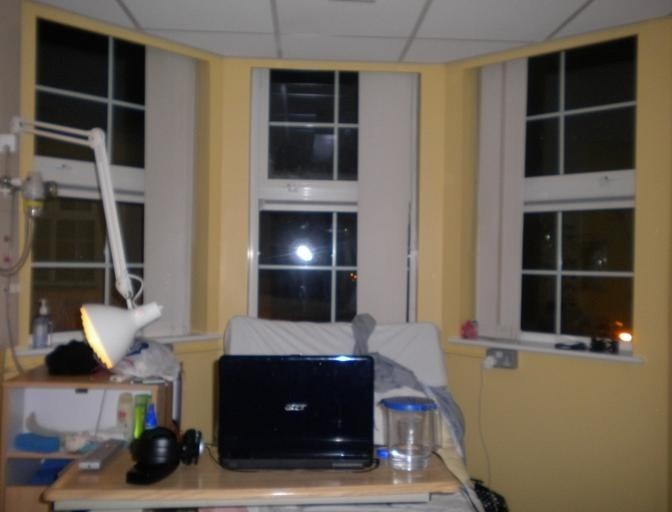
[380,396,441,475]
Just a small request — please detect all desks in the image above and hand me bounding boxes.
[42,441,464,509]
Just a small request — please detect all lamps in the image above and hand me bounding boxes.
[0,115,164,373]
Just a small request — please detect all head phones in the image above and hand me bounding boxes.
[179,428,204,464]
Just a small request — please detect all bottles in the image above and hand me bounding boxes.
[134,394,151,439]
[144,403,158,431]
[115,392,134,437]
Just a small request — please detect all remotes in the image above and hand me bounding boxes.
[78,438,125,471]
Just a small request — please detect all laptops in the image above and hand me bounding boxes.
[218,354,374,469]
